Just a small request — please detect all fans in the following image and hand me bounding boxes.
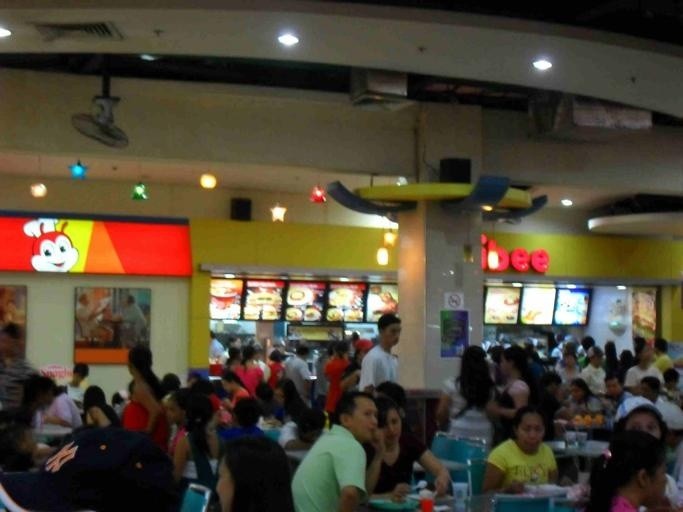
[70,55,132,151]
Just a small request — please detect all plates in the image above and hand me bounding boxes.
[326,308,363,322]
[328,289,353,306]
[286,307,320,322]
[286,288,311,305]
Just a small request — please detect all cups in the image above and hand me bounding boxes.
[451,481,468,512]
[210,280,237,309]
[560,431,586,447]
[420,493,433,512]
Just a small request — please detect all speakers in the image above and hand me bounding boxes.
[440,157,471,182]
[231,198,251,221]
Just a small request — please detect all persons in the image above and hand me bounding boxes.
[113,295,150,347]
[359,313,401,393]
[0,321,683,512]
[77,292,112,344]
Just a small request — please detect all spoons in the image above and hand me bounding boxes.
[411,480,427,491]
